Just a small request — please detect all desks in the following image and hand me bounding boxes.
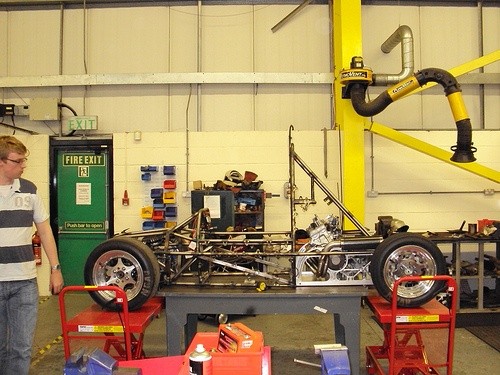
[154,276,370,375]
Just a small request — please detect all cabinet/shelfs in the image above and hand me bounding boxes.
[191,189,265,272]
[419,231,500,314]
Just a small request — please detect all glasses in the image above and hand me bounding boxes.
[6,158,28,163]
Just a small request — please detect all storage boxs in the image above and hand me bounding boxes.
[182,332,264,375]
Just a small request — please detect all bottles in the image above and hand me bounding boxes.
[188,343,213,375]
[483,224,498,236]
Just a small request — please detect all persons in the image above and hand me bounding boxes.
[0,135,64,375]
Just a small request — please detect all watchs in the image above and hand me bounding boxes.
[52,264,60,270]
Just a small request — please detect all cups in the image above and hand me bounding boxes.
[468,224,477,236]
